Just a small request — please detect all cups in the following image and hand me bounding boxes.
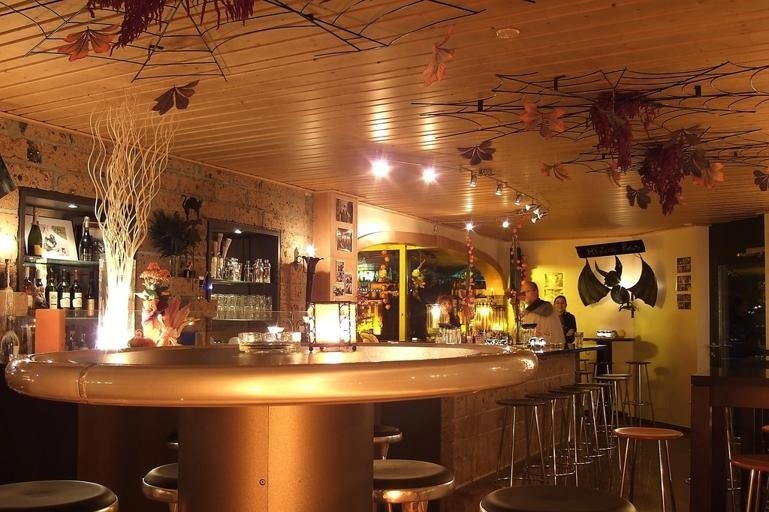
[439,328,462,344]
[210,294,273,320]
[574,331,584,346]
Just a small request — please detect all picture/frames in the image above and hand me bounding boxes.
[24,215,79,262]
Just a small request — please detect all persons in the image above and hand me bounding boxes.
[438,295,460,331]
[381,292,398,341]
[554,296,577,344]
[519,280,554,318]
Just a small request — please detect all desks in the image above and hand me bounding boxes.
[583,336,637,421]
[691,370,768,509]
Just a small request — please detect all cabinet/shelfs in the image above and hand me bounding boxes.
[17,186,137,351]
[206,218,281,346]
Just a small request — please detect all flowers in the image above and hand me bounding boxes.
[134,262,192,346]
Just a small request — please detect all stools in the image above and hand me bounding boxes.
[479,485,636,512]
[614,426,683,509]
[1,479,118,511]
[374,423,402,459]
[730,455,769,512]
[373,458,456,511]
[498,358,654,482]
[143,463,179,509]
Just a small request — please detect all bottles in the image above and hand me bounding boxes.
[210,258,272,283]
[77,215,93,262]
[27,206,42,257]
[25,267,96,317]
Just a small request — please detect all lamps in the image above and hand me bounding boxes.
[469,171,547,223]
[3,236,18,291]
[296,245,326,312]
